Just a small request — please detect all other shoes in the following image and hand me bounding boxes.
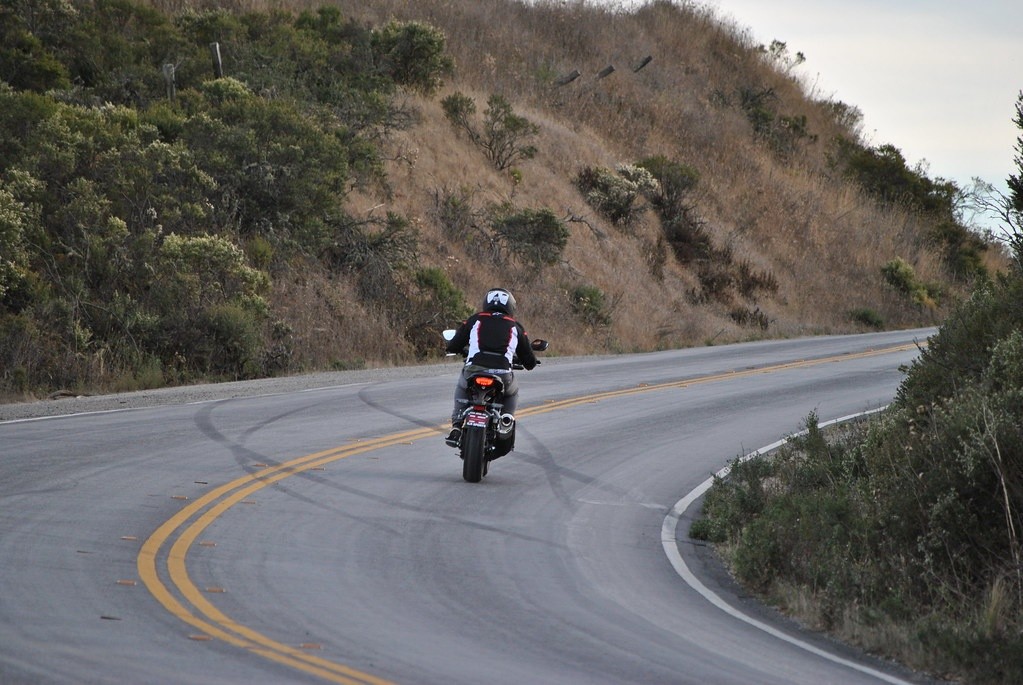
[446,423,462,447]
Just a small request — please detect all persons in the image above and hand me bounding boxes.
[446,288,538,447]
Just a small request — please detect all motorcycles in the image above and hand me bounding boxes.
[442,329,549,484]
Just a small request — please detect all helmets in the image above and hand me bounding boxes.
[483,288,516,317]
